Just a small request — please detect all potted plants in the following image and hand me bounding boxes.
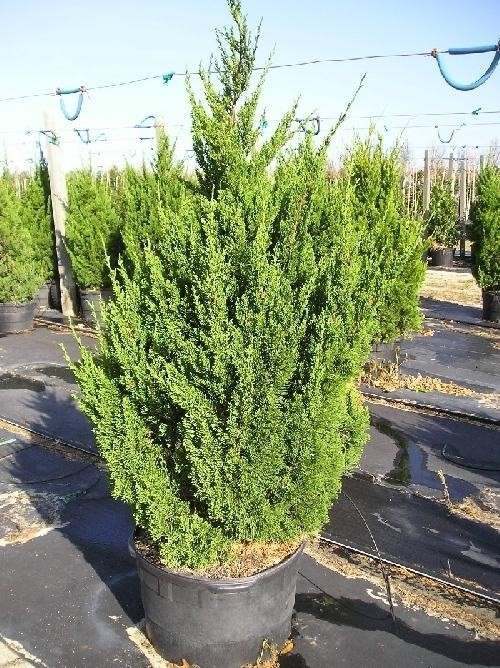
[465,164,499,322]
[424,167,464,267]
[60,1,425,667]
[1,124,184,334]
